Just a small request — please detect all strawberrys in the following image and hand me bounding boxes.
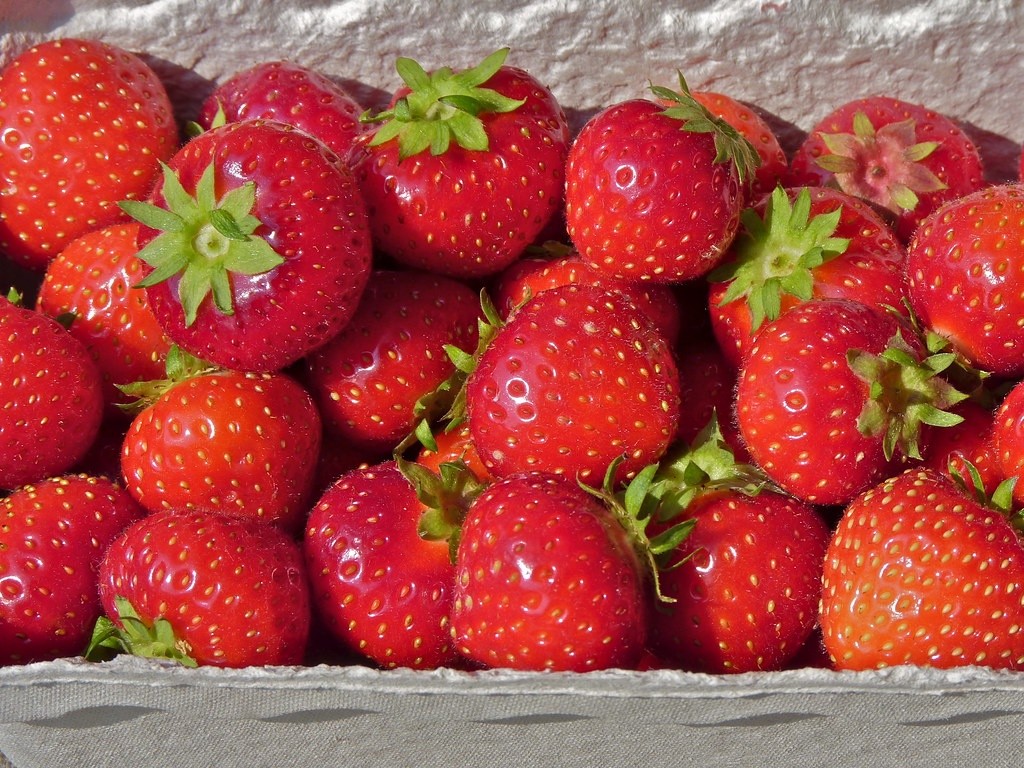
[0,38,1024,673]
